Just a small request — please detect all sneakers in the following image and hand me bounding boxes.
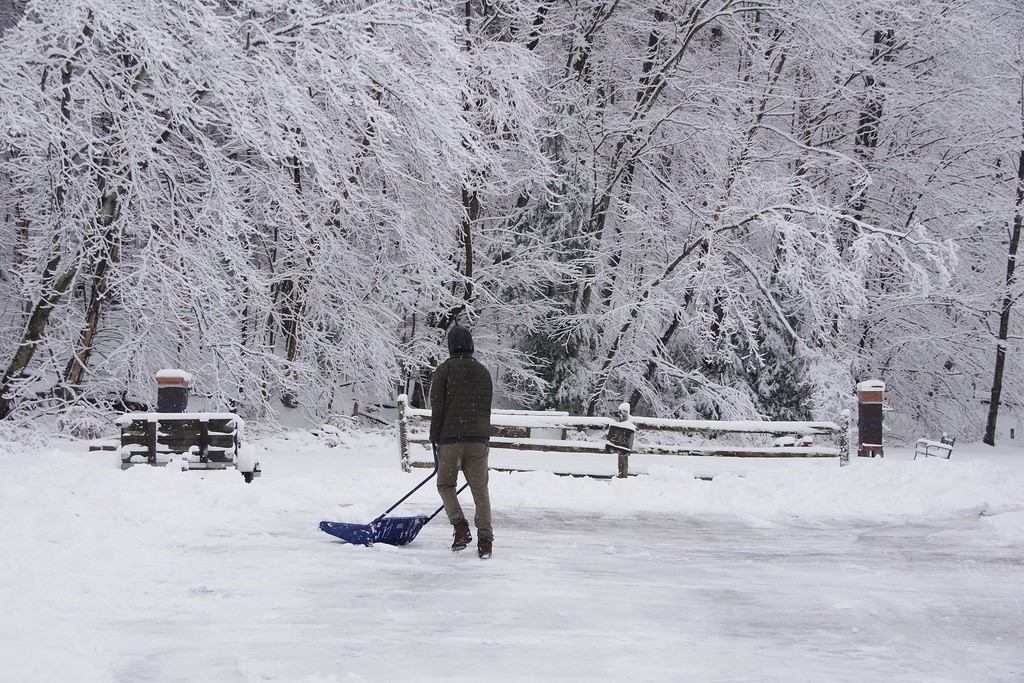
[451,524,472,551]
[477,539,492,559]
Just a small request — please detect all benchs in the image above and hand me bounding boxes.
[396,394,851,483]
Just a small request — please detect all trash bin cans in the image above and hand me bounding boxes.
[857,379,885,457]
[156,368,196,414]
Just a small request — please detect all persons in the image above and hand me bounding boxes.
[428,321,493,559]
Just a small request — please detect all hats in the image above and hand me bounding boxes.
[448,325,474,355]
